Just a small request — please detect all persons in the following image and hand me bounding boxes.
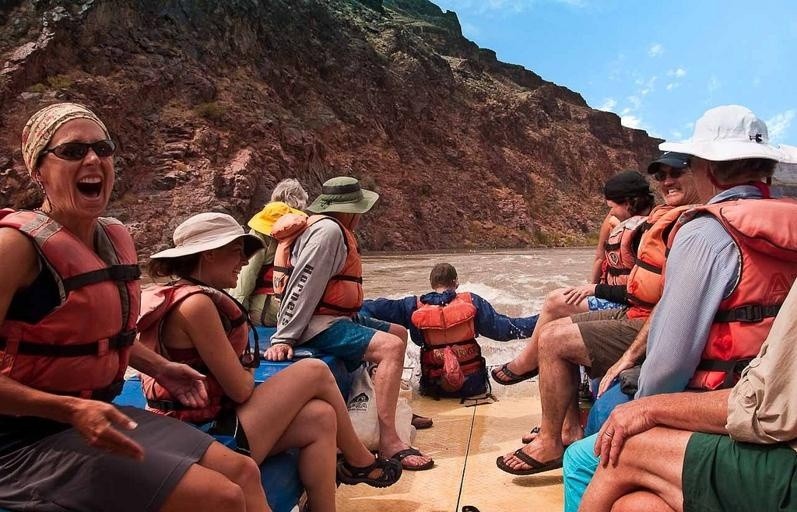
[139,213,402,511]
[229,179,308,328]
[496,153,703,475]
[577,276,797,512]
[564,105,797,512]
[492,170,654,398]
[264,176,434,471]
[357,263,540,399]
[0,103,272,512]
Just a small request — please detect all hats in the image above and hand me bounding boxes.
[307,177,379,213]
[150,213,267,259]
[659,105,797,163]
[647,151,692,174]
[248,202,307,236]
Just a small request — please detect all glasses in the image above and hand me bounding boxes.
[42,139,116,160]
[655,169,681,181]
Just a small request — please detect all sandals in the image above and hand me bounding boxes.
[336,454,402,488]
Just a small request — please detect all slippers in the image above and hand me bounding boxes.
[522,428,540,444]
[412,414,433,429]
[492,363,538,385]
[391,448,434,471]
[497,448,563,475]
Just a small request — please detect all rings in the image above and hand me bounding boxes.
[604,431,613,438]
